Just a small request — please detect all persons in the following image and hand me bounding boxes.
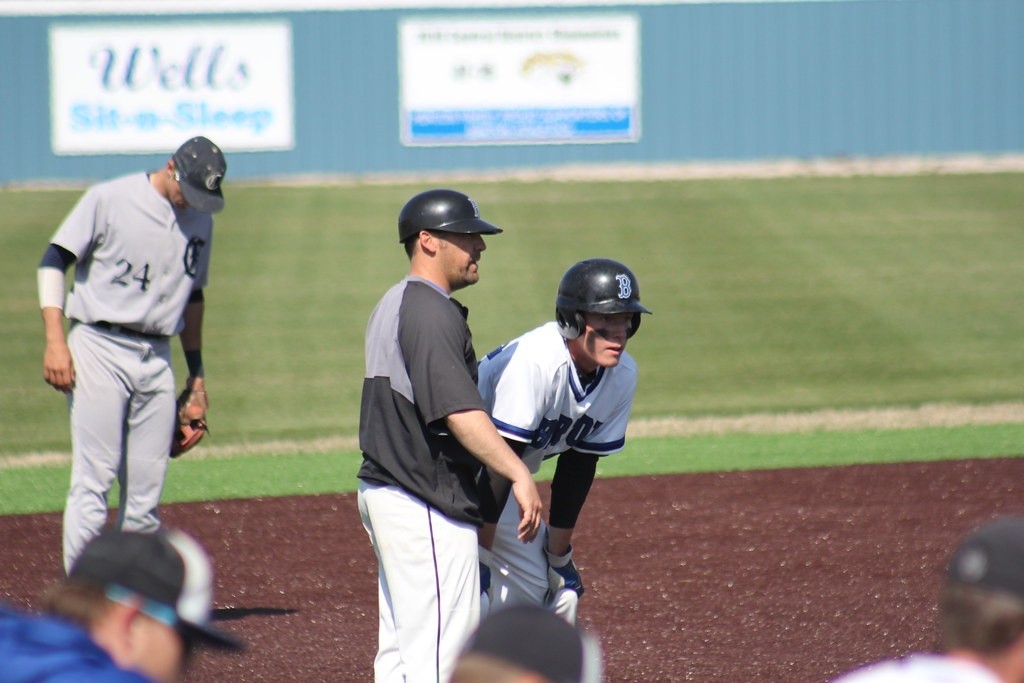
[34,136,230,579]
[472,259,654,620]
[3,527,252,683]
[446,605,586,683]
[827,515,1024,681]
[354,189,545,683]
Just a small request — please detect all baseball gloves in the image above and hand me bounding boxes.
[168,375,210,459]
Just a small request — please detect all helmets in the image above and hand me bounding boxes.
[397,188,504,243]
[556,259,653,341]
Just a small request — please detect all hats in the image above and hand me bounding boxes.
[67,530,247,652]
[172,136,227,213]
[943,516,1023,596]
[454,607,582,683]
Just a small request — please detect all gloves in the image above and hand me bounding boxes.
[478,544,492,597]
[542,541,584,602]
